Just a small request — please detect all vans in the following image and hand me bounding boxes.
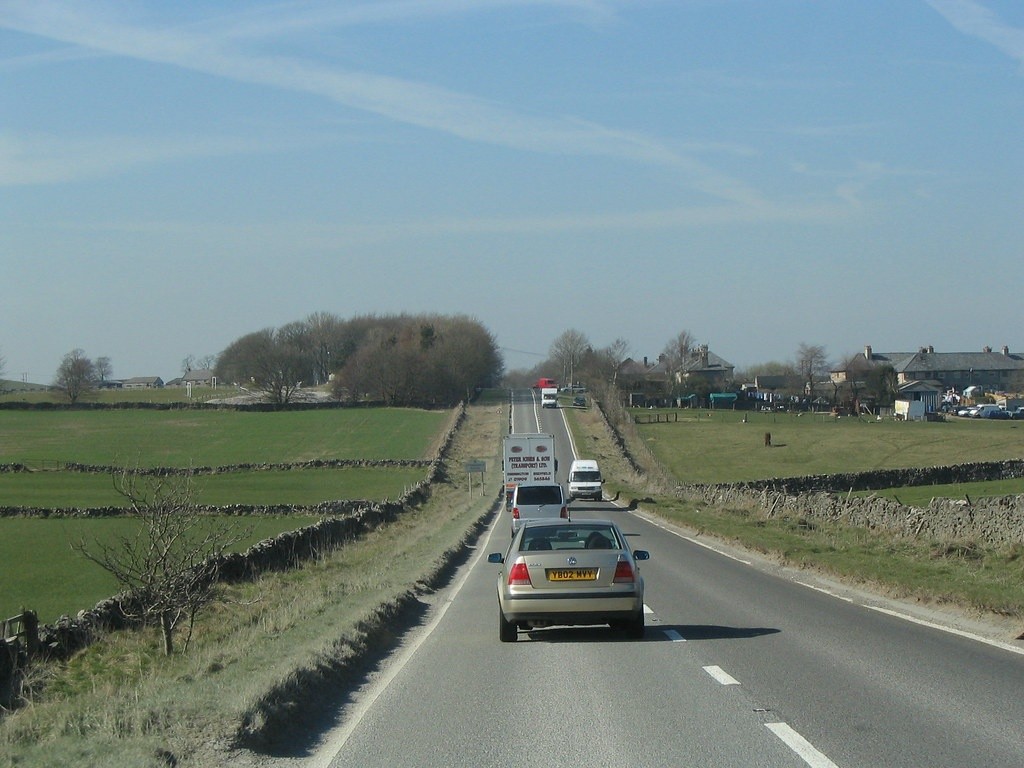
[511,482,567,536]
[567,459,605,501]
[968,404,999,418]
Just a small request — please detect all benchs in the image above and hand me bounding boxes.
[528,535,613,550]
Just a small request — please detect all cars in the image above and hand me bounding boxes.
[986,410,1008,420]
[533,384,538,388]
[958,407,974,416]
[572,396,585,406]
[561,385,586,393]
[948,407,964,416]
[486,518,650,642]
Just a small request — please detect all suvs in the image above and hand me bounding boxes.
[1011,407,1024,420]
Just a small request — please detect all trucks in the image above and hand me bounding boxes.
[502,432,557,511]
[541,388,558,408]
[539,378,556,389]
